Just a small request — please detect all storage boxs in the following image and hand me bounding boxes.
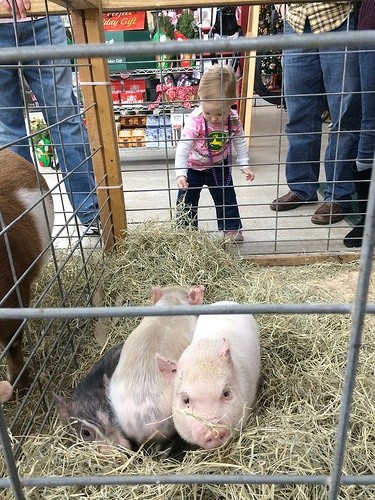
[104,29,156,70]
[110,77,150,105]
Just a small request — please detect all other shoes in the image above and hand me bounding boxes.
[82,219,104,236]
[223,230,244,244]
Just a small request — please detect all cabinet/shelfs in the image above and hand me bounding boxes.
[24,6,261,174]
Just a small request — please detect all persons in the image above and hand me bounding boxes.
[269,2,363,225]
[0,0,104,239]
[174,64,256,244]
[343,0,375,248]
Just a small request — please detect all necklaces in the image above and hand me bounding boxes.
[202,114,233,190]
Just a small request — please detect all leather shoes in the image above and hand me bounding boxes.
[311,196,350,224]
[270,192,319,211]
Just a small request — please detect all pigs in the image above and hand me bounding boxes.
[50,284,260,453]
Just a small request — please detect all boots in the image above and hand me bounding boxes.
[343,163,373,248]
